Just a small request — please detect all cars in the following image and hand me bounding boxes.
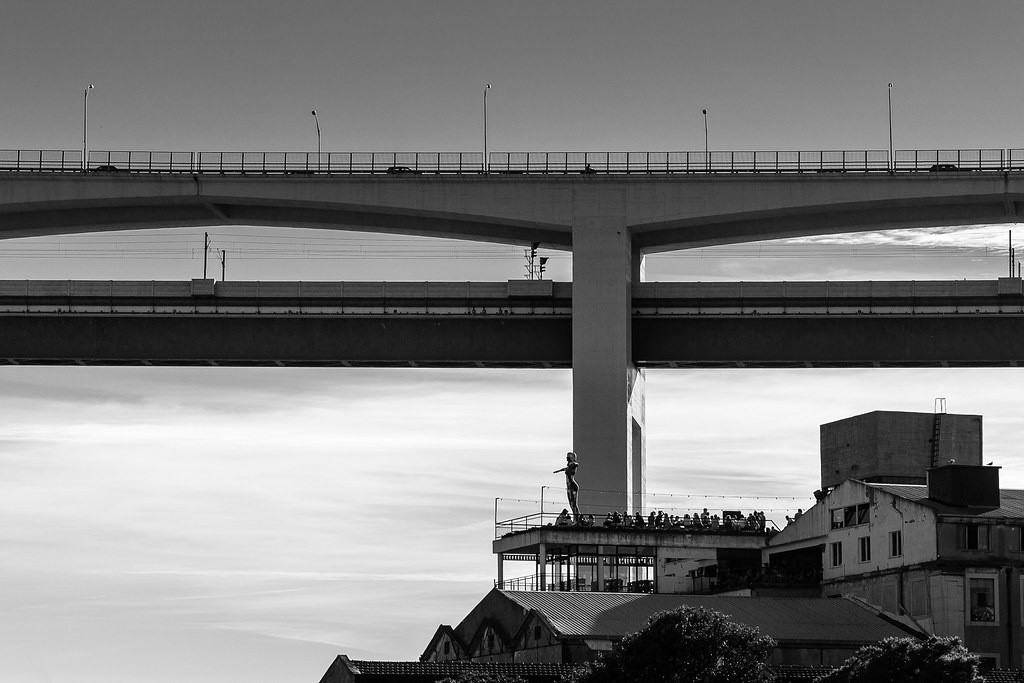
[929,163,973,172]
[95,165,119,173]
[387,166,421,175]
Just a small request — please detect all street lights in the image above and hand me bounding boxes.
[888,82,893,173]
[703,109,708,174]
[312,110,321,174]
[484,83,492,175]
[84,83,96,168]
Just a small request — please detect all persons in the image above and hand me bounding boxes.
[553,508,805,534]
[553,451,582,525]
